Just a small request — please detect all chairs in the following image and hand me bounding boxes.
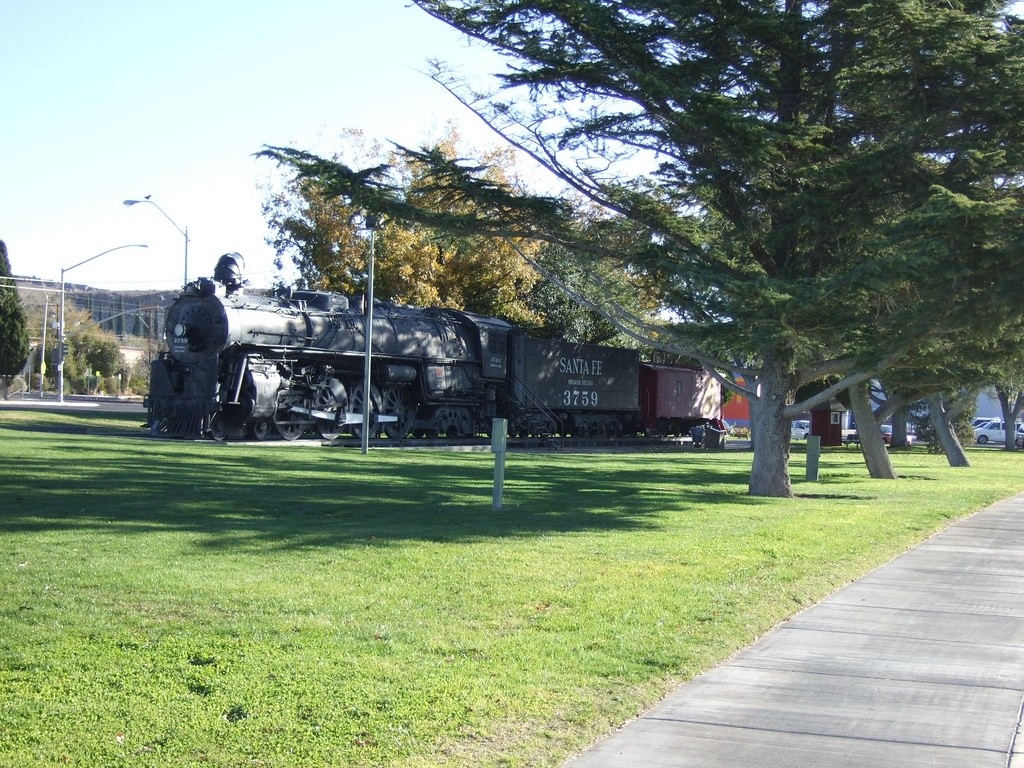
[841,433,853,449]
[853,434,861,450]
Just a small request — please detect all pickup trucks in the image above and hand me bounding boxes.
[791,420,810,440]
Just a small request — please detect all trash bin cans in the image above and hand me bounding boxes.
[704,426,725,450]
[691,427,705,442]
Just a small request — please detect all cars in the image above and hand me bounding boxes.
[880,424,893,444]
[972,417,1024,447]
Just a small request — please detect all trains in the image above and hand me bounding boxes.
[139,251,721,441]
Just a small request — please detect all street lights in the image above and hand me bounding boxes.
[57,244,147,405]
[122,198,190,289]
